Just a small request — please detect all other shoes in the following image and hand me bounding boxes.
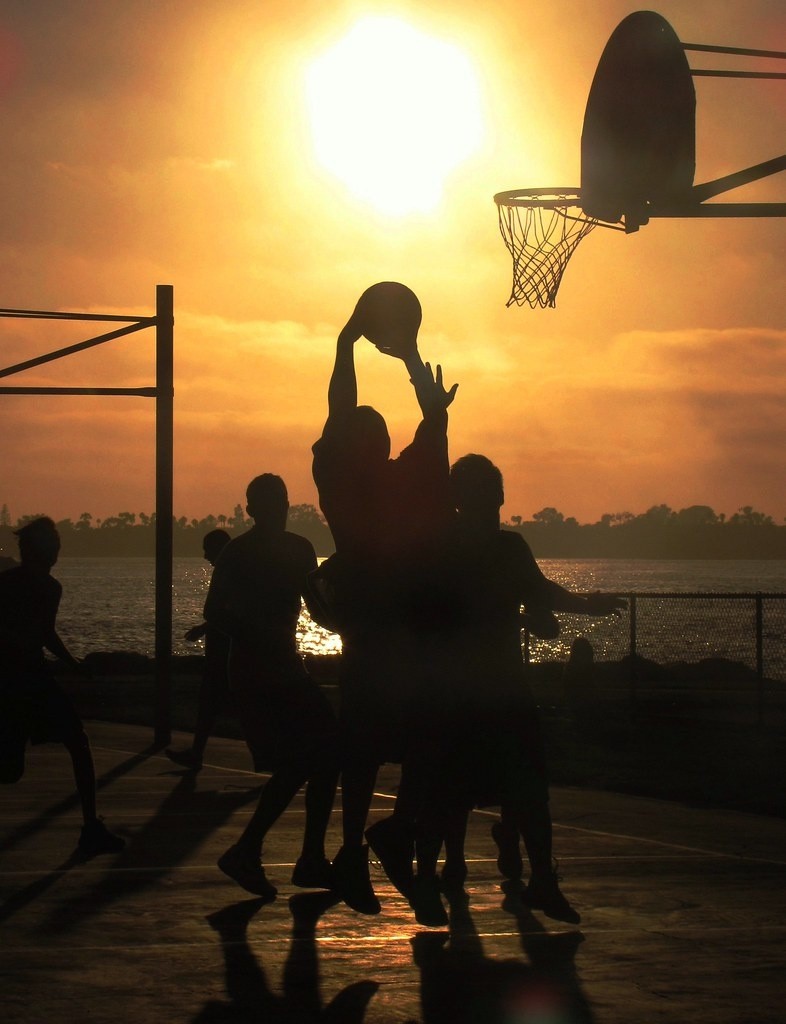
[521,876,581,924]
[491,820,524,881]
[327,843,381,914]
[217,846,278,898]
[79,814,125,855]
[441,863,471,902]
[292,856,334,888]
[165,747,204,770]
[363,817,416,897]
[408,873,450,926]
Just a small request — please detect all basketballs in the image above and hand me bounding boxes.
[356,282,424,348]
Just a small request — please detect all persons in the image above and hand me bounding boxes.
[0,518,129,856]
[165,293,629,924]
